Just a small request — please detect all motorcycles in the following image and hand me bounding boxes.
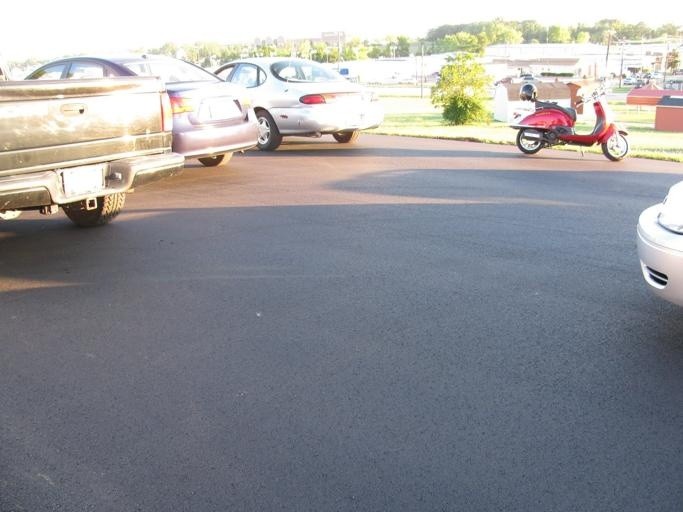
[509,83,630,162]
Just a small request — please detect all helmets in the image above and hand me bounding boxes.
[520,85,537,100]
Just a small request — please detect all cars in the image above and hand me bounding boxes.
[636,180,683,308]
